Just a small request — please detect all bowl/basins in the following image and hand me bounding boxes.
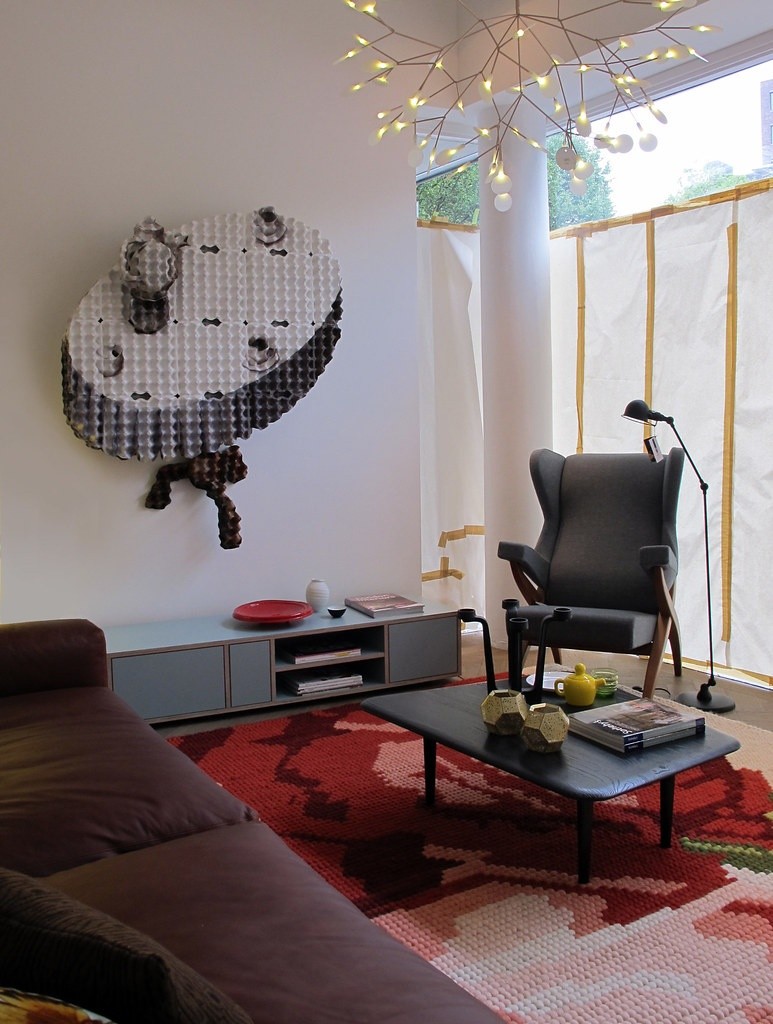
[328,605,347,618]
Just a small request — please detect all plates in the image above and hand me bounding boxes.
[526,670,574,692]
[233,600,314,623]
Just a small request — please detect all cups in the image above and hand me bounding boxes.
[592,667,619,696]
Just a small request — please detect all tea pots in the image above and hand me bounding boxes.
[554,664,606,707]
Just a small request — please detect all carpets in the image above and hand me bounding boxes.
[166,674,773,1024]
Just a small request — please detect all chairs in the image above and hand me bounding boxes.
[496,448,685,697]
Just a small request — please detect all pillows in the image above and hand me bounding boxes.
[0,868,255,1024]
[0,987,118,1024]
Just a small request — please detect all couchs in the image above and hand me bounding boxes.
[0,619,501,1024]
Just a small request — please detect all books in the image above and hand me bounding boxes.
[567,697,705,754]
[277,640,362,664]
[344,592,425,618]
[283,670,363,694]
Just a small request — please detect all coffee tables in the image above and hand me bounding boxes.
[362,680,741,886]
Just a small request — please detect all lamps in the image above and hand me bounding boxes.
[334,0,713,213]
[624,399,739,714]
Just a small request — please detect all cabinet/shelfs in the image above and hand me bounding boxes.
[106,597,461,726]
